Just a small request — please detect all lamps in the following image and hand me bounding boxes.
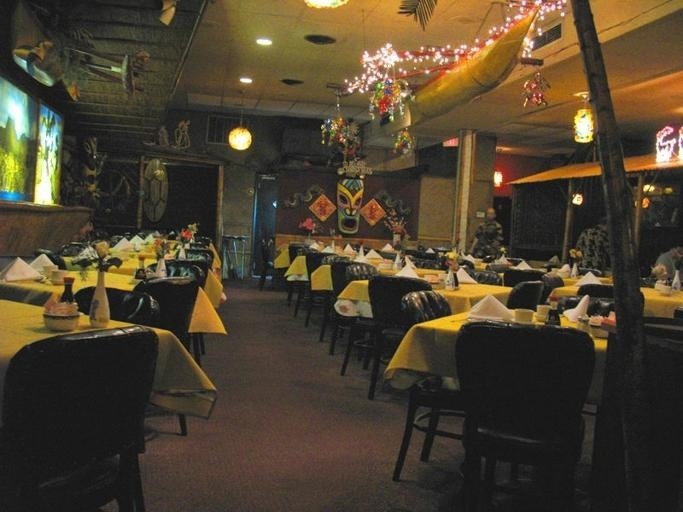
[227,108,253,152]
[571,91,594,144]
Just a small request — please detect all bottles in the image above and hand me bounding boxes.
[138,255,146,270]
[545,302,561,327]
[453,269,459,290]
[58,276,75,303]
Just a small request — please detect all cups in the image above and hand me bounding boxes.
[534,305,553,322]
[514,308,533,324]
[50,270,69,284]
[42,265,58,278]
[659,285,671,295]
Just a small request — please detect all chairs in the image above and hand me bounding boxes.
[287,243,310,307]
[319,255,353,344]
[476,271,502,285]
[73,285,161,328]
[329,256,353,355]
[293,248,319,317]
[133,276,199,435]
[367,275,433,401]
[146,261,205,369]
[503,268,545,287]
[391,290,464,481]
[60,242,83,256]
[183,249,212,268]
[0,326,158,512]
[339,262,378,377]
[542,272,564,303]
[304,252,338,327]
[577,284,644,311]
[34,247,67,271]
[454,318,596,512]
[507,281,545,310]
[485,264,509,272]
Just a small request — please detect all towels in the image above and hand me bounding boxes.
[425,247,436,253]
[496,253,511,264]
[29,253,58,273]
[152,230,161,237]
[455,267,476,284]
[382,242,394,252]
[557,263,572,277]
[113,237,132,250]
[516,260,533,269]
[465,254,475,264]
[565,272,565,273]
[322,245,334,253]
[309,242,319,251]
[467,294,514,322]
[343,244,354,252]
[571,262,579,277]
[577,271,602,287]
[549,255,559,263]
[563,295,590,323]
[145,234,153,244]
[1,256,42,281]
[365,248,382,259]
[129,234,144,244]
[395,263,418,278]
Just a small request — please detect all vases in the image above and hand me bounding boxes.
[156,258,167,279]
[178,248,186,261]
[571,262,579,279]
[445,267,455,291]
[89,272,110,327]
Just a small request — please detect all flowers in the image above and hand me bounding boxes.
[672,247,683,270]
[93,242,122,272]
[568,248,583,263]
[152,239,167,258]
[181,231,192,248]
[445,251,459,273]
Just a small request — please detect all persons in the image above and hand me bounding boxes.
[655,239,683,279]
[467,209,503,255]
[576,216,609,270]
[484,225,501,263]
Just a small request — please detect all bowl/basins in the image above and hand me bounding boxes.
[42,312,80,333]
